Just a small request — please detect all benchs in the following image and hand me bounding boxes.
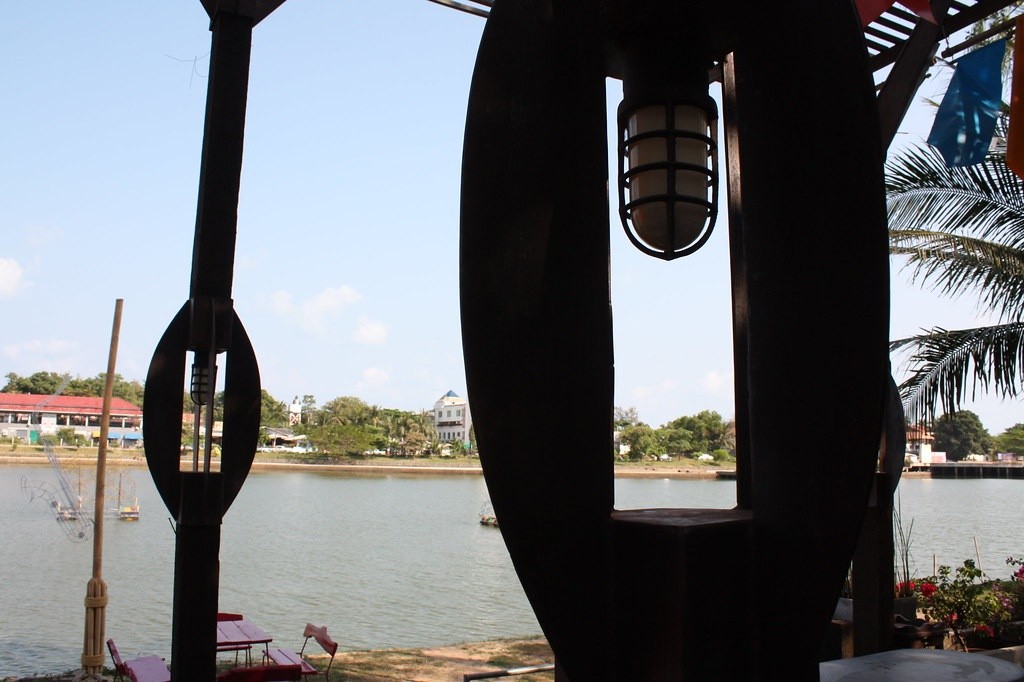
[217,612,252,668]
[107,638,171,682]
[262,623,338,682]
[217,664,304,682]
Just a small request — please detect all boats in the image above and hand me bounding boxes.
[48,460,140,521]
[716,469,737,477]
[479,501,498,527]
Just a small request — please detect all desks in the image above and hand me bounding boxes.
[217,620,272,669]
